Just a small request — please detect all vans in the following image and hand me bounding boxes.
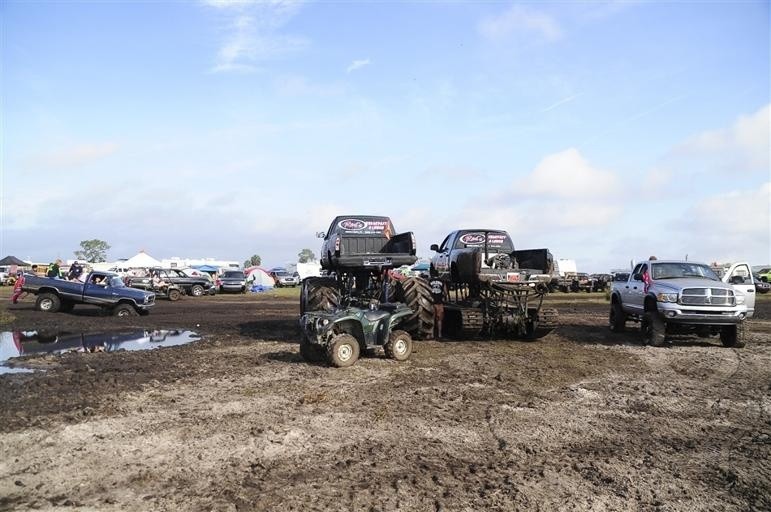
[219,271,246,293]
[270,272,299,287]
[555,272,614,293]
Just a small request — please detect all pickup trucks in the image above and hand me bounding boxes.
[125,268,216,297]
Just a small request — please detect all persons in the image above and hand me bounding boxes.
[212,268,224,293]
[29,265,38,275]
[125,267,167,287]
[68,261,82,280]
[45,260,66,279]
[429,269,448,337]
[95,276,107,285]
[633,270,650,294]
[12,271,25,302]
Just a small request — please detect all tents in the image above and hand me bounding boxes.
[243,266,276,293]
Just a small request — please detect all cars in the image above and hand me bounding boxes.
[753,278,770,293]
[754,268,771,282]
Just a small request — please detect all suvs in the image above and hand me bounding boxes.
[299,299,415,368]
